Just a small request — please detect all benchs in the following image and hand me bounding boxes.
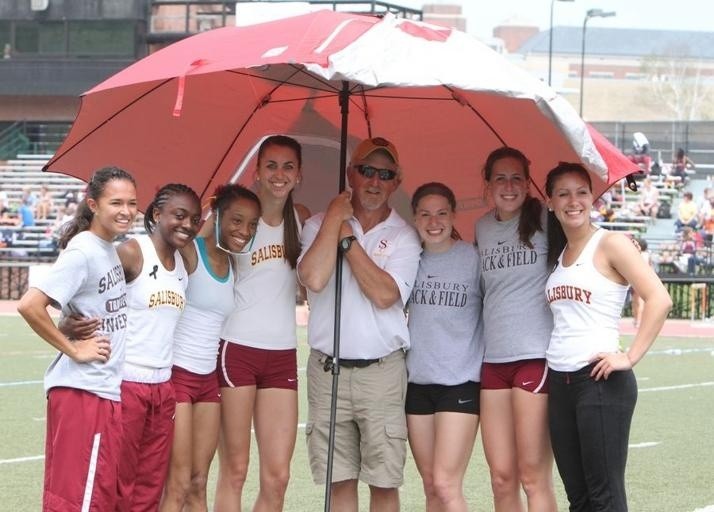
[588,163,695,227]
[1,154,147,262]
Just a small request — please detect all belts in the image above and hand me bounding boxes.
[325,356,379,369]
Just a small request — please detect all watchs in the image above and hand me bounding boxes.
[339,235,357,252]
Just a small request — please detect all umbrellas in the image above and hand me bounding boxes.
[42,10,643,512]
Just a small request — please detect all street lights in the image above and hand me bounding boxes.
[578,8,617,116]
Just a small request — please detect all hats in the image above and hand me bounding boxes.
[352,137,398,163]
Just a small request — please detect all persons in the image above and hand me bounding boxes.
[214,136,311,512]
[1,181,85,257]
[545,158,673,512]
[585,133,714,273]
[158,183,261,512]
[401,183,486,512]
[295,135,421,512]
[631,240,652,325]
[472,146,562,512]
[16,169,137,512]
[59,184,201,512]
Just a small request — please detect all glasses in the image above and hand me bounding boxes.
[353,164,397,180]
[215,208,256,255]
